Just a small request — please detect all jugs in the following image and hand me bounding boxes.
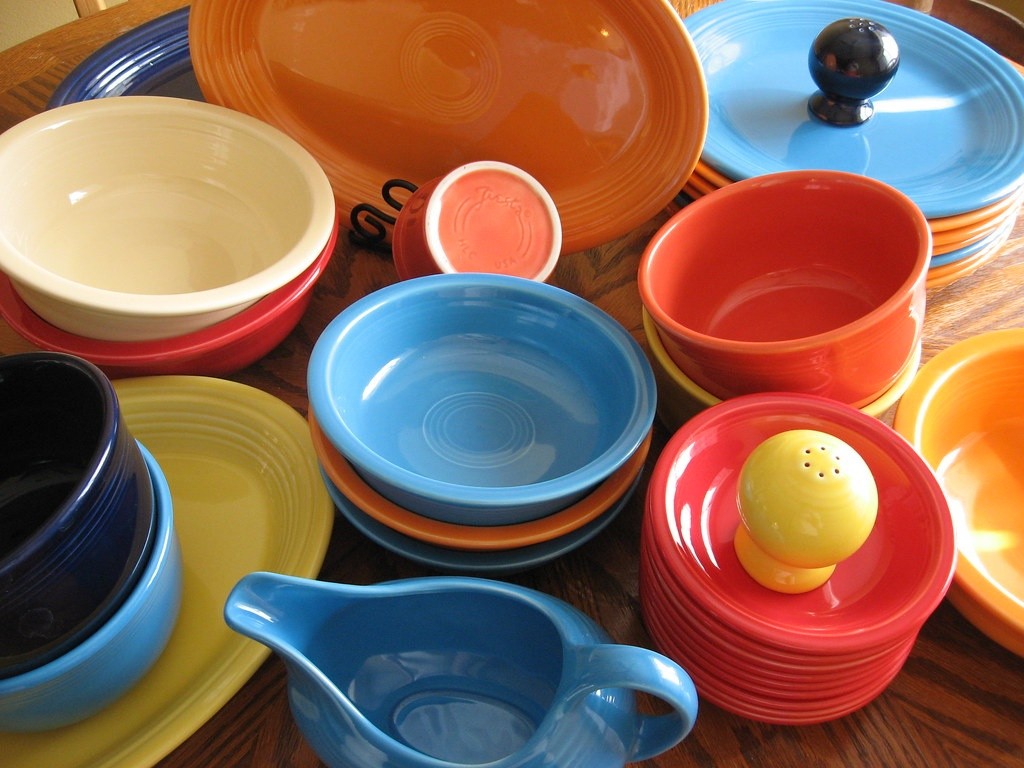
[224,570,698,768]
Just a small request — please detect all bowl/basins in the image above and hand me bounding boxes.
[317,463,644,570]
[636,169,932,409]
[0,352,157,680]
[307,271,657,525]
[0,439,184,733]
[0,95,336,340]
[0,210,340,374]
[307,403,653,550]
[642,305,923,408]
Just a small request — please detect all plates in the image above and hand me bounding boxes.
[893,328,1024,656]
[641,392,959,727]
[666,0,1024,288]
[47,5,205,110]
[187,0,708,256]
[0,375,335,768]
